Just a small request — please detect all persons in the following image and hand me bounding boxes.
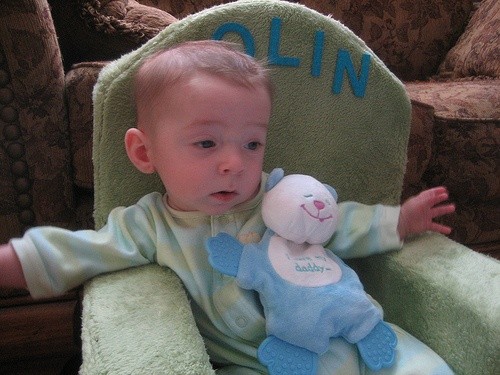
[0,37,462,375]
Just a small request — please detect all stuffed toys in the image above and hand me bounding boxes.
[205,166,398,375]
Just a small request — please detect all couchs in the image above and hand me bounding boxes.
[0,1,500,300]
[80,1,500,375]
